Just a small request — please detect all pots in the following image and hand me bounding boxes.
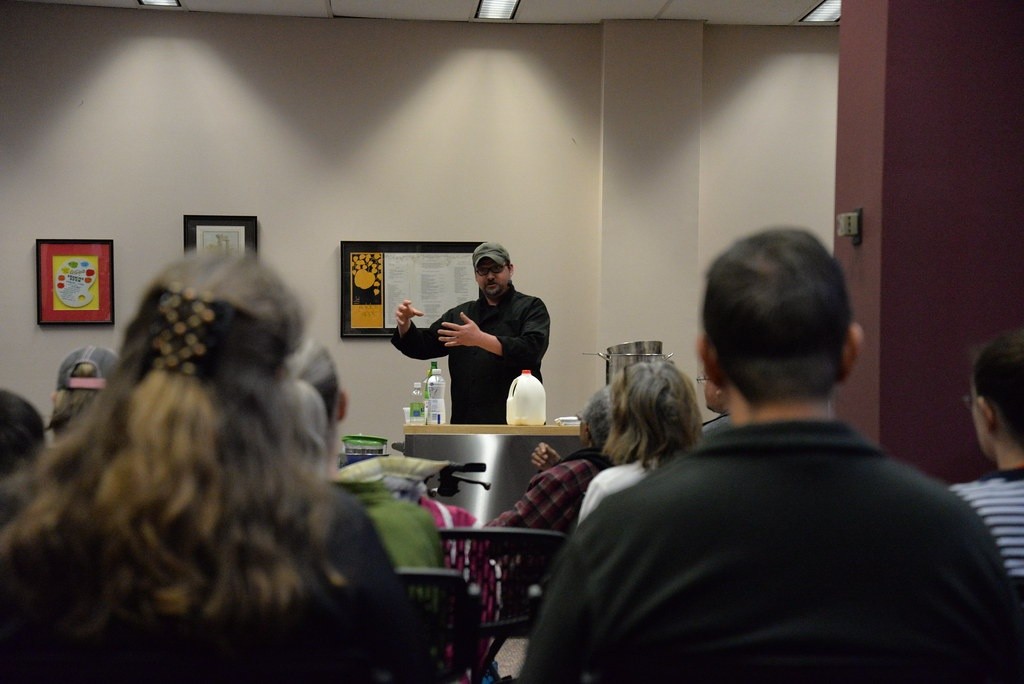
[582,352,675,385]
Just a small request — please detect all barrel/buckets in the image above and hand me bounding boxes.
[343,436,388,454]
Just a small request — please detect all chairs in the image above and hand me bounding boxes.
[397,528,573,684]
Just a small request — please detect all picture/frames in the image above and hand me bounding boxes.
[36,239,115,326]
[340,240,486,337]
[183,214,257,255]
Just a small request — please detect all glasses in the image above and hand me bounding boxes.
[961,388,999,412]
[575,411,593,433]
[474,263,509,277]
[696,374,712,385]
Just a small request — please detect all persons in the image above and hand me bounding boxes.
[390,242,551,425]
[477,359,740,622]
[948,330,1024,579]
[515,227,1024,684]
[0,242,480,684]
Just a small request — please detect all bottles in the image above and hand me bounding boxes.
[410,382,426,425]
[506,370,547,426]
[423,360,438,424]
[428,368,446,424]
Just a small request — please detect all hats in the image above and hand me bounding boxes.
[56,346,120,390]
[472,242,511,269]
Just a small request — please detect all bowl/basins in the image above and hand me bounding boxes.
[402,407,428,424]
[607,341,662,354]
